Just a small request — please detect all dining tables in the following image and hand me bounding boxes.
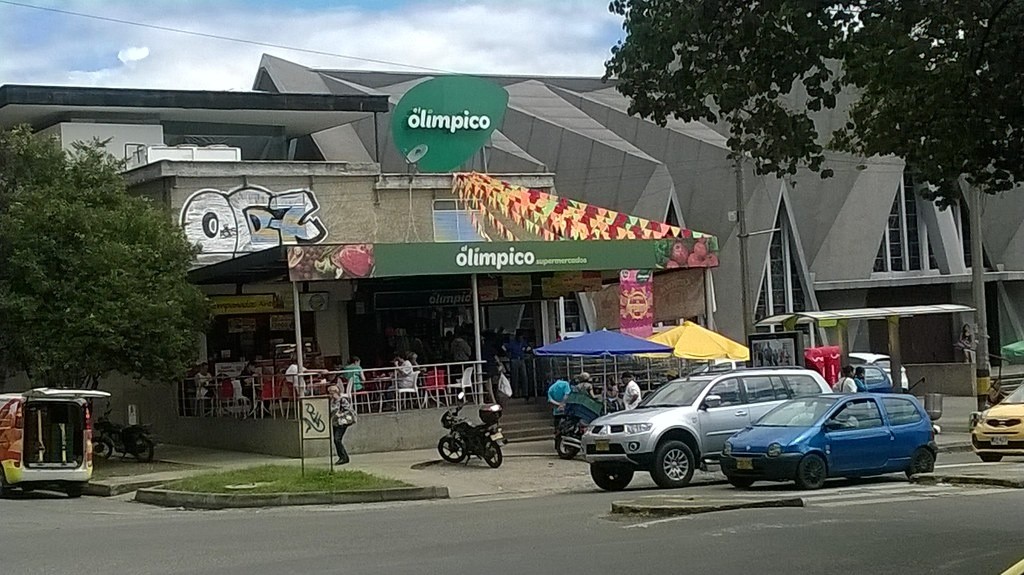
[203,383,233,419]
[243,384,272,419]
[370,378,396,413]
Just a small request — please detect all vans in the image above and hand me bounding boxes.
[0,387,112,496]
[582,366,834,492]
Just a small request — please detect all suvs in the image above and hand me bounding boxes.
[848,352,908,392]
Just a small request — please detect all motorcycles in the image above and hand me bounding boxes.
[554,380,623,459]
[438,392,506,468]
[92,403,154,463]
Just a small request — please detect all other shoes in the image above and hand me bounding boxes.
[336,457,350,465]
[383,406,393,411]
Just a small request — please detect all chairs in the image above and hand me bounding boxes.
[448,367,476,405]
[183,364,396,420]
[423,371,449,409]
[393,370,422,410]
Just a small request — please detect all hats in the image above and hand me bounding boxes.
[620,372,631,379]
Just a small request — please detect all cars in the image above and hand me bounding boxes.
[852,366,891,392]
[721,393,938,489]
[971,380,1024,462]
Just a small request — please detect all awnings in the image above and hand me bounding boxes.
[179,236,721,286]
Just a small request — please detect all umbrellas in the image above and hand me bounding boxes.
[633,320,751,391]
[535,329,674,413]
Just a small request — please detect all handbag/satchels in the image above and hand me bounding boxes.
[498,372,513,397]
[334,398,354,426]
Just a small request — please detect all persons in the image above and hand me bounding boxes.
[602,376,619,415]
[328,386,351,465]
[576,372,596,399]
[547,375,571,432]
[959,323,975,363]
[620,371,642,410]
[832,408,859,429]
[195,322,533,416]
[667,369,679,381]
[832,366,868,392]
